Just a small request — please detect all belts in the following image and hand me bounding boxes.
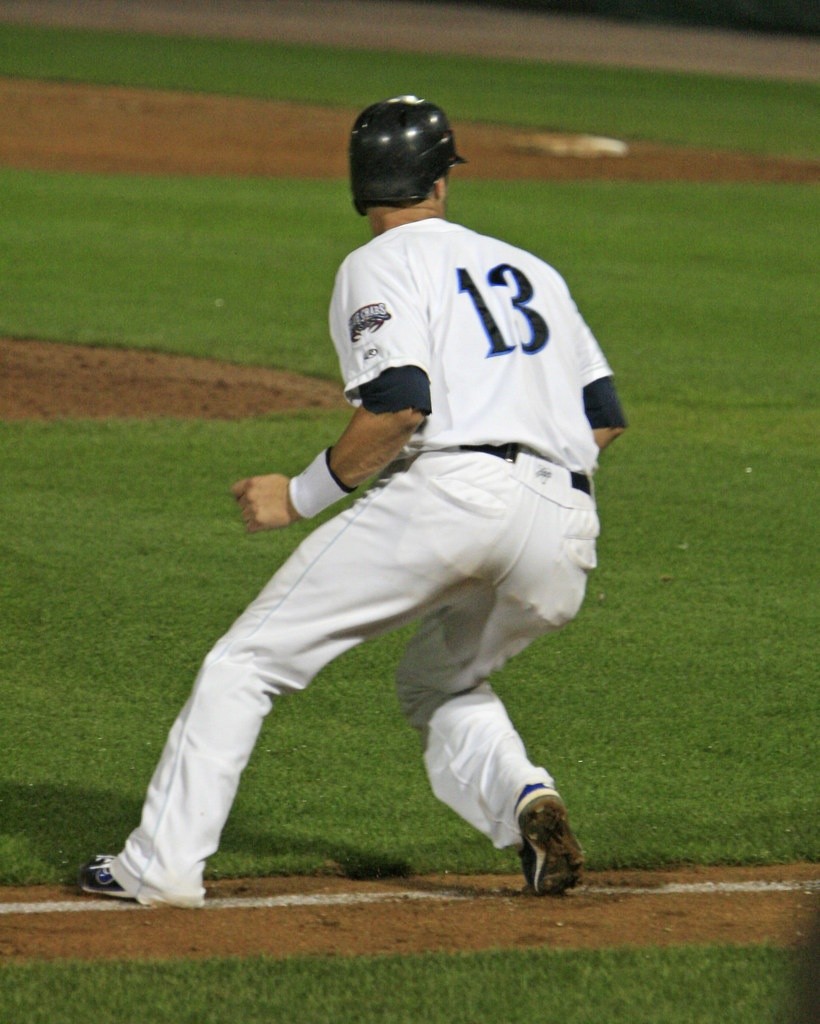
[459,441,590,494]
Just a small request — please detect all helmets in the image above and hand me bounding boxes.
[349,94,467,215]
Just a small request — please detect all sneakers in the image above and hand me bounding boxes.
[514,781,584,896]
[77,854,137,899]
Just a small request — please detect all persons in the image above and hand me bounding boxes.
[79,93,628,909]
